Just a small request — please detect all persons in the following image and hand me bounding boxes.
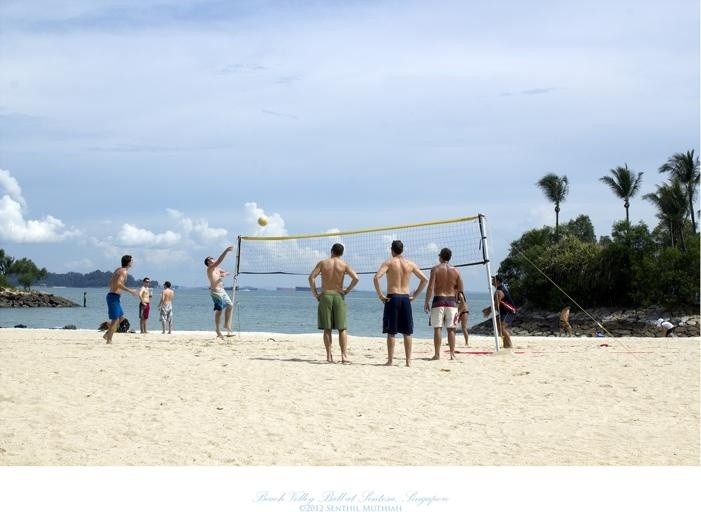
[138,277,151,334]
[660,320,675,338]
[424,247,470,361]
[372,238,429,367]
[157,280,175,334]
[482,274,516,348]
[307,242,360,364]
[203,244,235,340]
[101,254,138,344]
[558,304,573,337]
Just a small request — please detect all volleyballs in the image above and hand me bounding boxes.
[258,216,268,226]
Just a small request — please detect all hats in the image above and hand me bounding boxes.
[491,275,503,282]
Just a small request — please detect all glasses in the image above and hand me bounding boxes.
[145,281,150,283]
